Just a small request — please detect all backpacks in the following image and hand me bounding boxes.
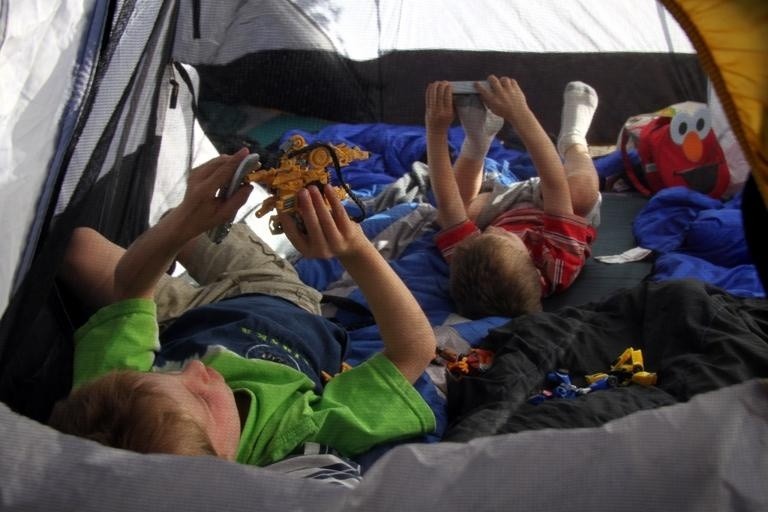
[616,102,732,200]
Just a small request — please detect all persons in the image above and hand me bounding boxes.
[425,75,603,321]
[59,147,438,461]
